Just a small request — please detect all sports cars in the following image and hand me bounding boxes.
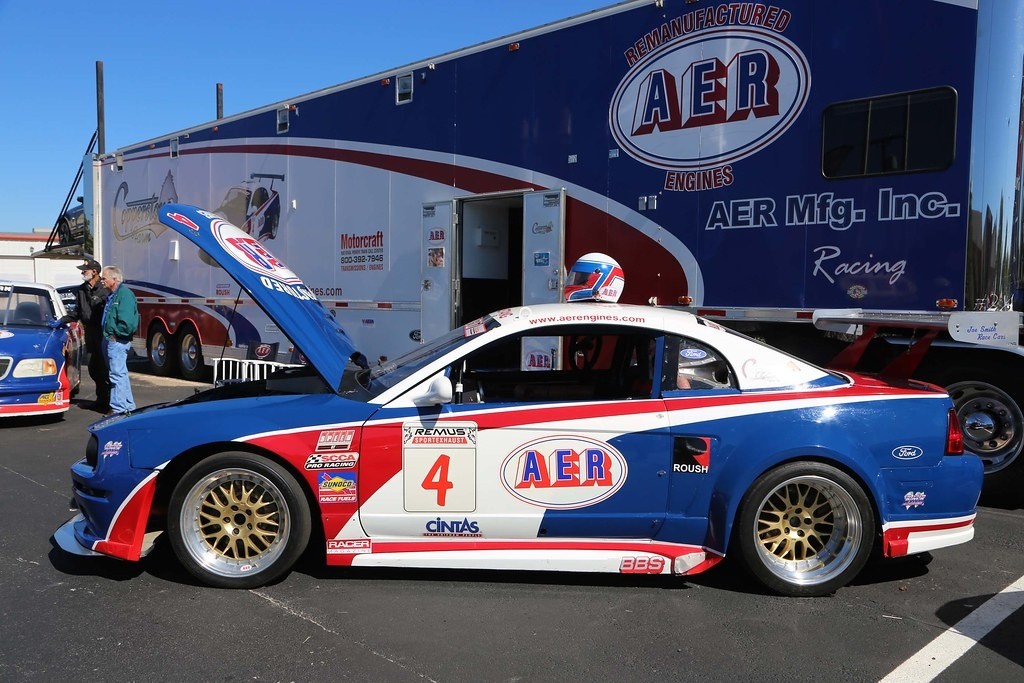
[51,204,986,601]
[0,278,84,419]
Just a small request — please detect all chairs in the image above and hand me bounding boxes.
[14,301,41,325]
[624,336,655,399]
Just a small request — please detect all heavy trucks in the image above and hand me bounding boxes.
[91,2,1024,502]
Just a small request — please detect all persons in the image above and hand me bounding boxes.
[59,259,112,414]
[99,266,139,418]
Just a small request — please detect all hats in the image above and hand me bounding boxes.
[76,260,101,273]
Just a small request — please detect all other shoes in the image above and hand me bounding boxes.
[103,409,116,418]
[90,402,108,414]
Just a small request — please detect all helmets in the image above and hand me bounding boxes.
[565,253,625,304]
